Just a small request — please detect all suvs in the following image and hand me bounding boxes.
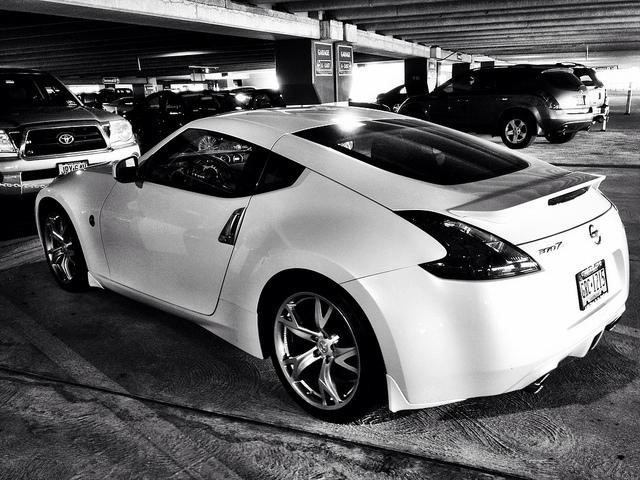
[0,65,144,211]
[348,59,611,146]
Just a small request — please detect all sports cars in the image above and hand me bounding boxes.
[35,103,631,424]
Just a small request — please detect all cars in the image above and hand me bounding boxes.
[76,84,285,149]
